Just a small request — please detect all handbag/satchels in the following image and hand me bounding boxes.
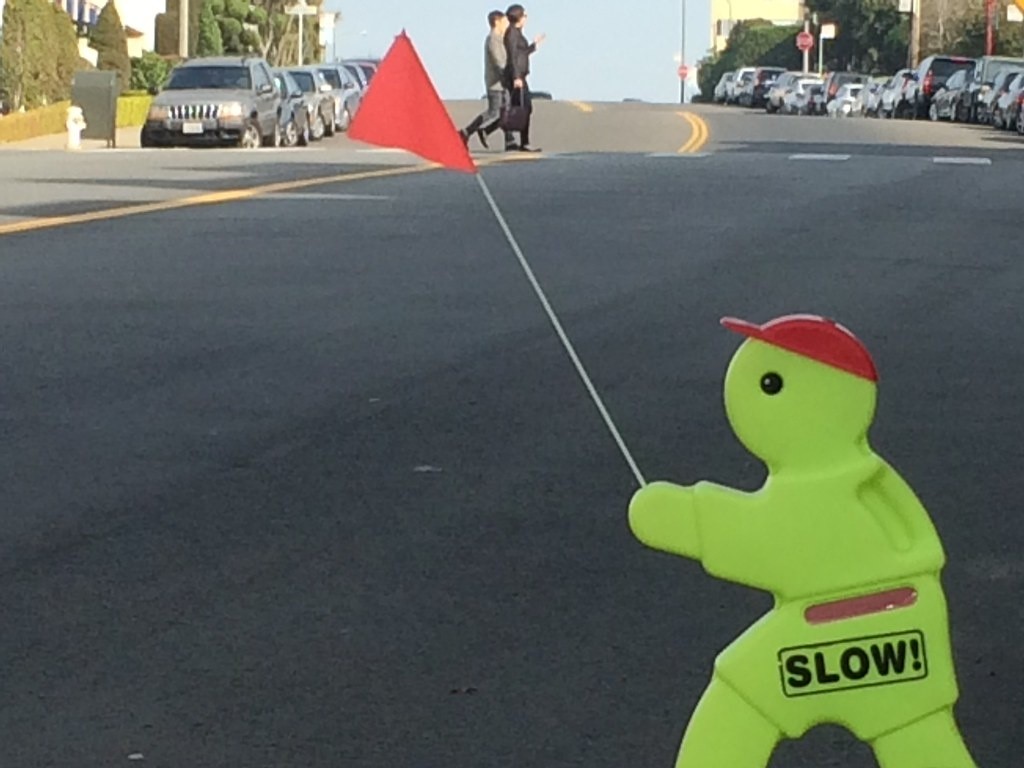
[500,87,530,131]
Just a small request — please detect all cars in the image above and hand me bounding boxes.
[315,64,361,130]
[287,65,336,139]
[271,67,310,147]
[344,59,381,93]
[140,55,282,148]
[715,54,1024,136]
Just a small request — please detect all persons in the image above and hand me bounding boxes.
[458,4,546,152]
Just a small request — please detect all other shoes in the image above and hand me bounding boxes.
[478,128,488,148]
[460,129,469,152]
[518,147,542,152]
[506,143,520,150]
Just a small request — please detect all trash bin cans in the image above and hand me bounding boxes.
[69,69,122,141]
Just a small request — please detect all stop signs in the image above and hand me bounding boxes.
[796,32,813,51]
[677,64,687,78]
